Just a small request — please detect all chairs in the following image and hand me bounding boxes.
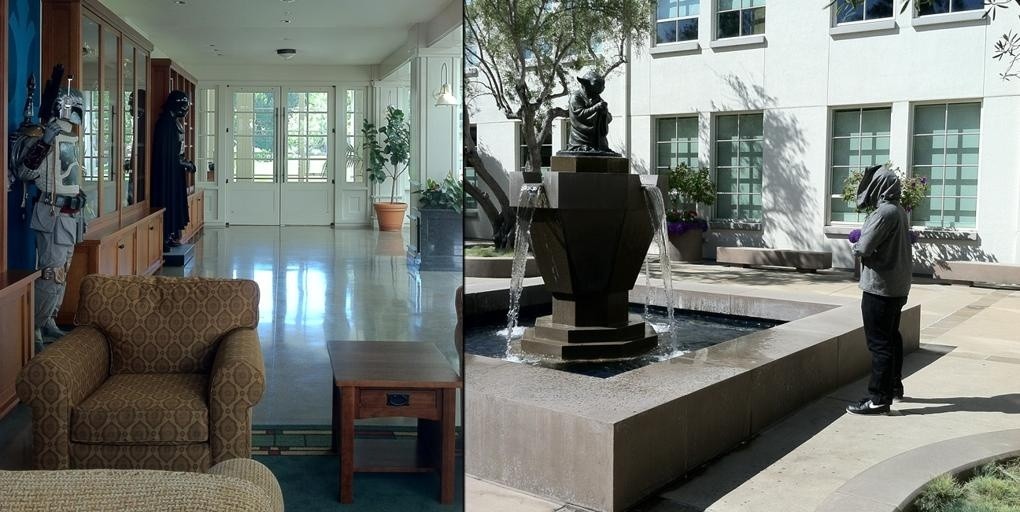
[16,273,265,471]
[0,458,284,512]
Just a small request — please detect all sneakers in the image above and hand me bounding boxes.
[35,319,65,354]
[845,399,891,416]
[893,395,904,399]
[163,238,182,252]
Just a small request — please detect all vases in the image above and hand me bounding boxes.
[853,255,860,281]
[664,227,704,264]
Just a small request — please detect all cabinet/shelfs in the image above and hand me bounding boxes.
[0,0,205,420]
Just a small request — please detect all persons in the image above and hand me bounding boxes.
[150,89,198,254]
[123,88,145,204]
[16,87,90,354]
[566,71,612,152]
[845,165,913,415]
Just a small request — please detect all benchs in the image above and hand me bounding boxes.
[933,261,1020,287]
[716,246,832,273]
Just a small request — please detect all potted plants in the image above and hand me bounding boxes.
[361,105,410,232]
[410,171,463,272]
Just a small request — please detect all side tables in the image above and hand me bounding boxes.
[326,341,463,503]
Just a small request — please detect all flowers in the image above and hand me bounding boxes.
[663,161,716,237]
[840,160,928,243]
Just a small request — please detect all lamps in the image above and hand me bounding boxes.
[433,62,459,106]
[277,49,296,59]
